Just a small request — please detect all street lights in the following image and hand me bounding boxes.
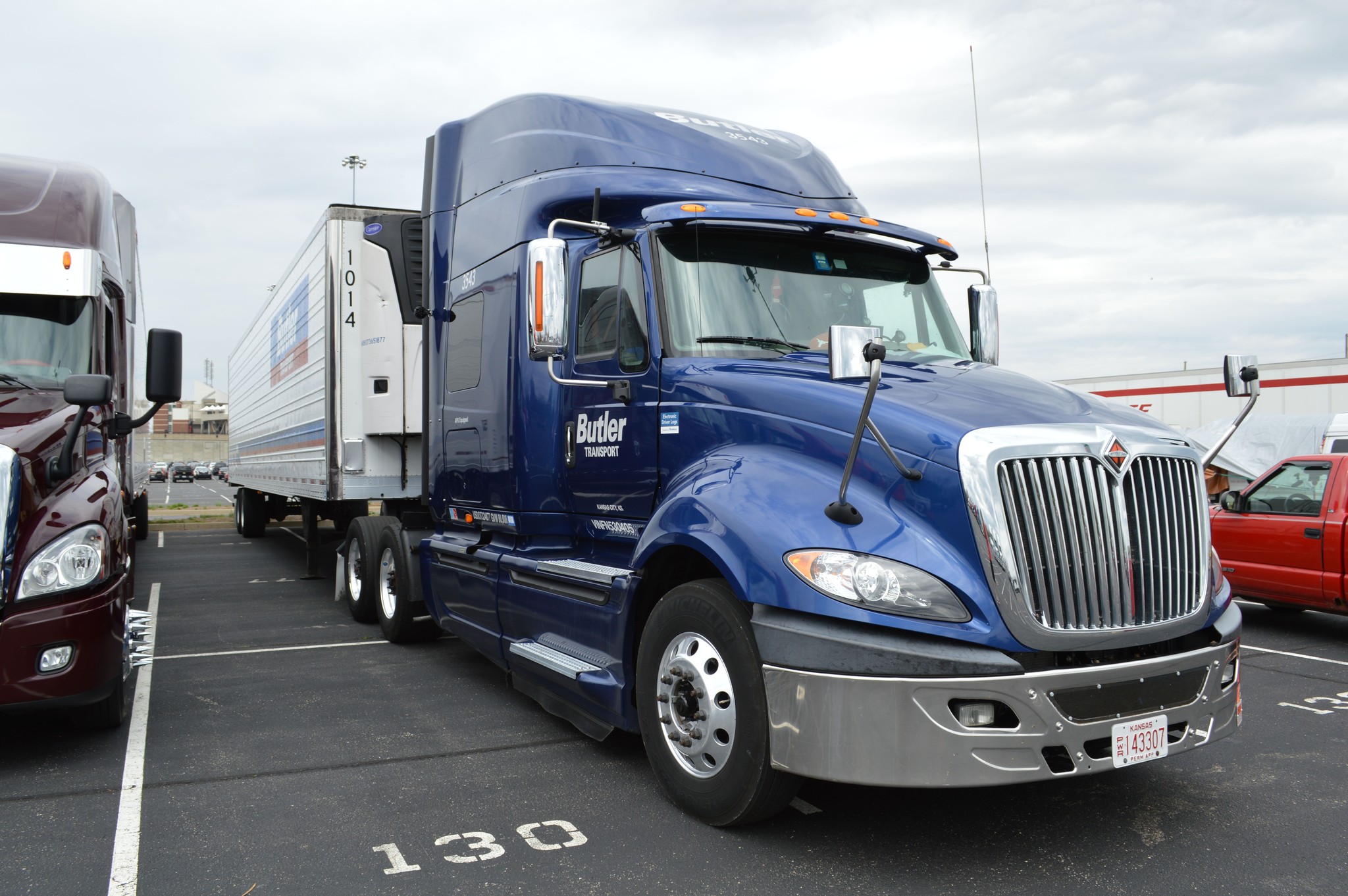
[340,155,367,204]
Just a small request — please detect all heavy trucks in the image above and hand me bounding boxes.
[0,156,183,725]
[226,92,1262,827]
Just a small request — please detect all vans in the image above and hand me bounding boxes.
[1316,414,1348,506]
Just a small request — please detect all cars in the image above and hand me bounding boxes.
[1207,454,1348,619]
[145,457,225,481]
